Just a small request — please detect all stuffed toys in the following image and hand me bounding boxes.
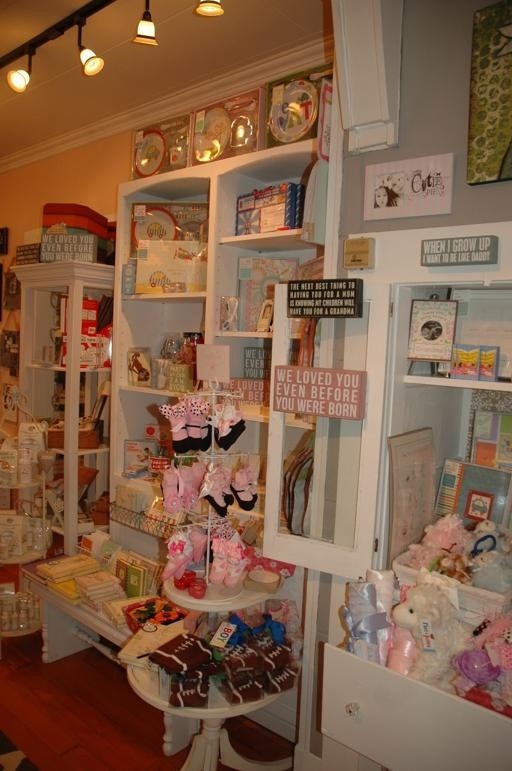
[388,514,512,718]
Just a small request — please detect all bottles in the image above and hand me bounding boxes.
[180,330,204,364]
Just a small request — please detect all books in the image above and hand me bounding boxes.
[37,530,188,670]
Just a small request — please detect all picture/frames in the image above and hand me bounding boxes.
[464,489,495,523]
[364,151,454,220]
[406,299,459,362]
[387,427,434,570]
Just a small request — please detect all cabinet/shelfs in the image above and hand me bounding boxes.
[28,137,318,759]
[0,384,47,638]
[9,259,115,557]
[125,379,298,770]
[262,219,512,770]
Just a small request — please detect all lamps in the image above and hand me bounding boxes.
[7,50,35,95]
[132,0,158,46]
[74,18,105,77]
[195,0,225,17]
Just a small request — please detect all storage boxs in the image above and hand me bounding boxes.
[43,428,100,449]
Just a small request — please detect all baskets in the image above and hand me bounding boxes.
[393,551,512,619]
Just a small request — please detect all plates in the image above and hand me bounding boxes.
[193,107,231,162]
[131,207,180,249]
[269,79,319,143]
[133,127,168,178]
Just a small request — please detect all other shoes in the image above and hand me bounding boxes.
[159,419,258,599]
